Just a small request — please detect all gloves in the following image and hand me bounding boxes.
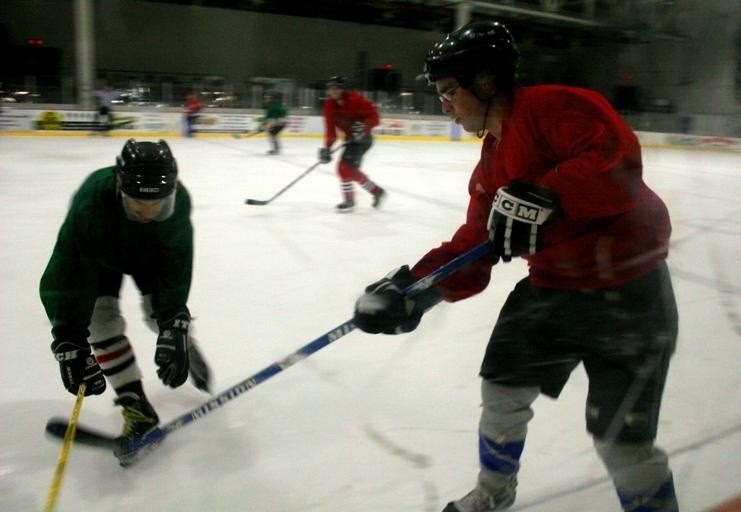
[154,310,189,389]
[487,179,557,266]
[319,147,330,162]
[51,334,104,395]
[353,264,442,335]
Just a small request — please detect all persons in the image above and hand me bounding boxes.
[88,85,123,125]
[180,89,202,139]
[318,74,386,213]
[255,90,287,155]
[354,15,683,511]
[39,139,211,469]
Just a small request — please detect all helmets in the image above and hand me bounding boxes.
[117,137,176,221]
[424,22,518,108]
[324,75,345,90]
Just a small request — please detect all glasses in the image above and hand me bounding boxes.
[438,82,459,101]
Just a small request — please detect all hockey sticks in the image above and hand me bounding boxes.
[47,239,494,453]
[245,142,347,205]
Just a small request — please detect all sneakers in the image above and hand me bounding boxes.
[442,467,518,512]
[112,388,159,456]
[337,187,385,208]
